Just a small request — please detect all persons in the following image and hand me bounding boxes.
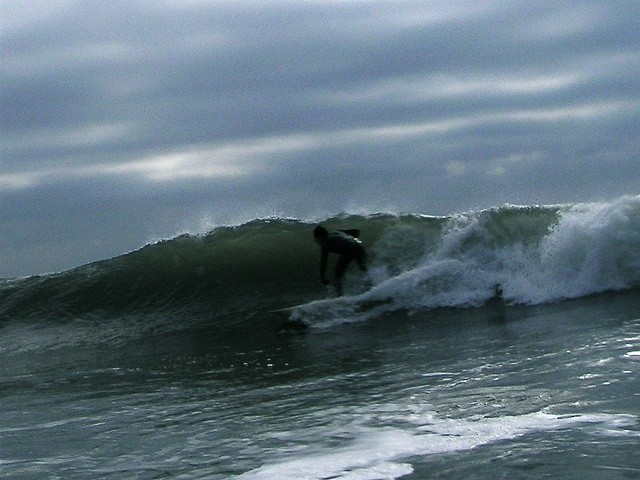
[313,227,373,297]
